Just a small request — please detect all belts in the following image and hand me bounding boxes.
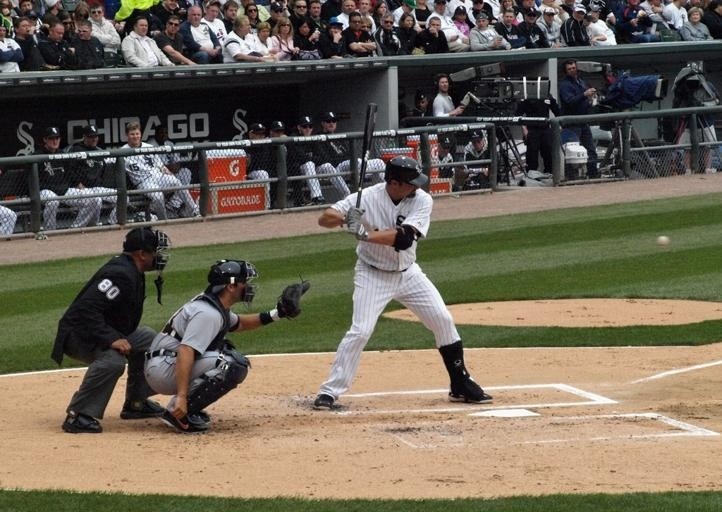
[144,349,178,359]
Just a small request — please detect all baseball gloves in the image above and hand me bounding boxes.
[278,279,310,320]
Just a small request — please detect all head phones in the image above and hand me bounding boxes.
[433,73,451,86]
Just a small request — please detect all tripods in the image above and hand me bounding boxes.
[601,121,657,179]
[668,116,722,169]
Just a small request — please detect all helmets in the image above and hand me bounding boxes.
[204,259,257,302]
[122,226,171,270]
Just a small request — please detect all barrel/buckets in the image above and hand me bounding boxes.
[406,133,440,178]
[379,146,414,165]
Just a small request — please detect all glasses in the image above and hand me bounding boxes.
[77,29,90,33]
[91,9,104,14]
[294,3,307,9]
[324,118,337,123]
[168,20,179,27]
[246,8,259,14]
[300,123,313,130]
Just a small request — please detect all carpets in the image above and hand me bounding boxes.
[2,489,720,512]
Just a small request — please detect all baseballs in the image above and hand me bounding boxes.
[658,236,669,246]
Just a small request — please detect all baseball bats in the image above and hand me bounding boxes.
[356,103,378,208]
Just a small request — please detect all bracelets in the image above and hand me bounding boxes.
[258,312,274,326]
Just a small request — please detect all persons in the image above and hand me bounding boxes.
[313,154,493,411]
[143,259,310,434]
[50,225,171,433]
[1,1,722,237]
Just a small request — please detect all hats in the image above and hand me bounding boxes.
[43,122,65,142]
[383,155,430,189]
[572,4,587,15]
[468,129,485,141]
[269,1,284,9]
[523,6,556,15]
[328,16,343,25]
[439,136,453,149]
[454,5,488,20]
[249,109,337,134]
[82,123,103,136]
[205,0,222,8]
[400,0,419,9]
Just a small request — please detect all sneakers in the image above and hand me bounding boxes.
[120,397,166,419]
[300,196,327,205]
[162,411,207,432]
[443,375,492,403]
[313,392,337,410]
[61,411,102,435]
